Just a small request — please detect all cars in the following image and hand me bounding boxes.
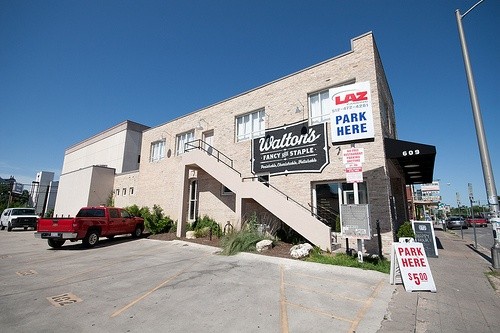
[445,212,491,229]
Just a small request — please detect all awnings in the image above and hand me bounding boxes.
[384,136,436,185]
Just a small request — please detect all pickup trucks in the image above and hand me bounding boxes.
[34,206,144,248]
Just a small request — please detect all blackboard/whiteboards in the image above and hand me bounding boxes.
[412,220,438,257]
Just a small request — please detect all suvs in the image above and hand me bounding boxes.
[0,207,40,232]
